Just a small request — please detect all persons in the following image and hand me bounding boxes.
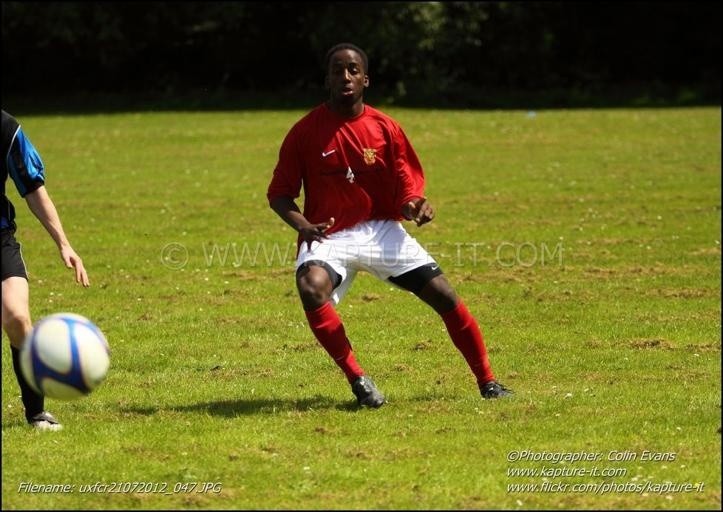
[0,111,91,434]
[265,42,516,409]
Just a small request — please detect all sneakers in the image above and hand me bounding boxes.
[478,379,514,400]
[28,408,64,434]
[351,373,384,408]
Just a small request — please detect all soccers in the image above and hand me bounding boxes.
[19,313,111,401]
